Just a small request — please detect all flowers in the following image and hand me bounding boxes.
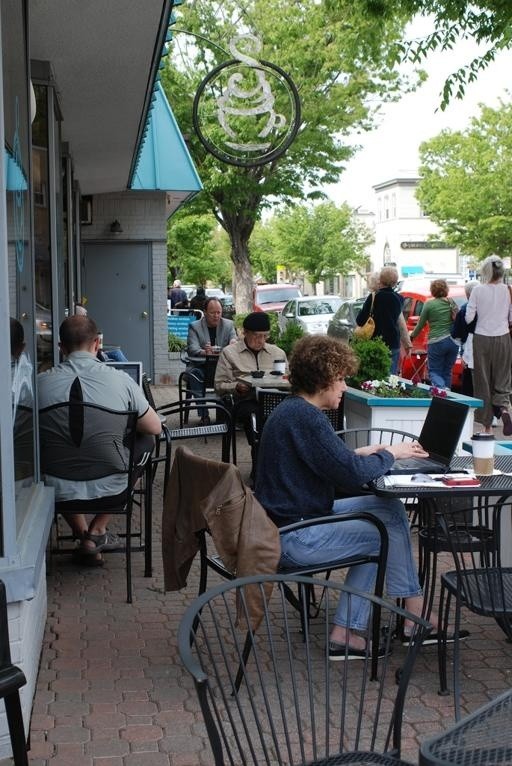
[362,373,450,399]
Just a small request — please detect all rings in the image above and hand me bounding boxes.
[415,448,417,453]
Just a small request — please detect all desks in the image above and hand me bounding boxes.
[51,408,166,578]
[418,686,512,764]
[240,370,290,390]
[186,352,227,424]
[360,454,512,642]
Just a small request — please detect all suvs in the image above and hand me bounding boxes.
[253,284,306,319]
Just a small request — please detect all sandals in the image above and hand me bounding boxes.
[76,528,120,567]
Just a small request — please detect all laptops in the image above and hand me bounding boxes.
[387,394,471,474]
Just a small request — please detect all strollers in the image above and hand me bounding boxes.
[400,333,431,384]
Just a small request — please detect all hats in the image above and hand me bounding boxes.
[243,312,270,332]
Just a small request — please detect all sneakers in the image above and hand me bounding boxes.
[473,430,496,435]
[502,412,512,436]
[403,625,469,647]
[325,638,392,661]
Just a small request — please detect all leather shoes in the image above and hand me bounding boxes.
[197,415,211,426]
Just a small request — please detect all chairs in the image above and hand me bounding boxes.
[172,571,436,766]
[138,375,237,462]
[171,352,231,443]
[251,386,346,449]
[428,491,512,696]
[164,445,389,695]
[177,343,203,420]
[308,430,426,604]
[37,398,173,606]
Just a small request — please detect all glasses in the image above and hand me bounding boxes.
[336,374,348,382]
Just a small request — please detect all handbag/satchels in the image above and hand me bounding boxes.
[451,307,459,320]
[354,291,375,339]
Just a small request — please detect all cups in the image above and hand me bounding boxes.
[471,432,496,475]
[273,360,287,373]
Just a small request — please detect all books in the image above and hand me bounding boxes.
[383,472,481,489]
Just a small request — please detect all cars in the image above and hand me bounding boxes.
[281,296,341,338]
[328,298,367,339]
[394,286,470,392]
[178,281,233,301]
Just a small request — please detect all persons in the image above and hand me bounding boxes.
[214,311,290,491]
[186,294,233,429]
[190,287,211,319]
[33,317,163,569]
[169,279,189,315]
[74,304,129,363]
[10,317,37,458]
[355,254,512,440]
[250,335,469,662]
